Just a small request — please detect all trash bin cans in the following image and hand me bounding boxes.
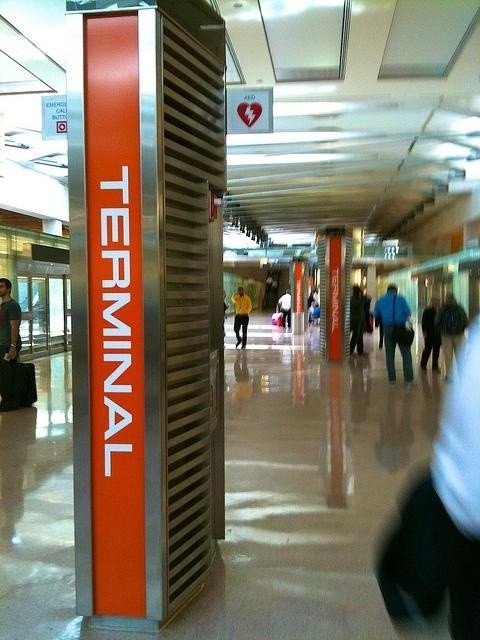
[291,312,305,335]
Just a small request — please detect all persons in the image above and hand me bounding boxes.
[349,285,384,360]
[434,291,469,384]
[374,319,480,639]
[278,289,291,329]
[307,287,321,327]
[231,286,253,350]
[420,296,442,374]
[373,285,415,385]
[0,278,23,365]
[223,290,231,338]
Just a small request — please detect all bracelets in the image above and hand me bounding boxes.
[10,344,17,350]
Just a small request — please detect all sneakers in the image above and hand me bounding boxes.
[390,379,413,385]
[421,363,441,372]
[236,341,245,348]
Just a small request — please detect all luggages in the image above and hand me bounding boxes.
[0,361,37,413]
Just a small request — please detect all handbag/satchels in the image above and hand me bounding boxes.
[394,325,415,344]
